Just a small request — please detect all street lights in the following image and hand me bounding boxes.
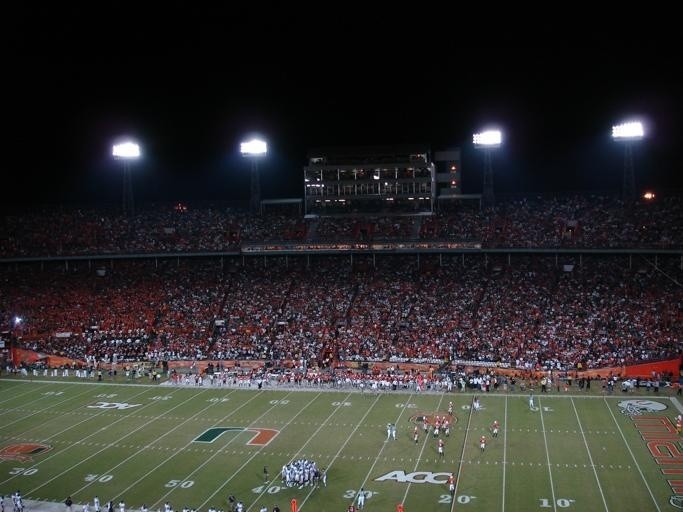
[472,129,503,206]
[612,120,645,201]
[113,143,142,216]
[239,140,268,211]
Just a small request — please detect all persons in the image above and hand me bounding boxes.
[674,413,682,433]
[280,457,326,489]
[1,199,683,394]
[0,489,298,512]
[263,464,270,484]
[383,395,539,455]
[447,475,455,493]
[356,487,364,510]
